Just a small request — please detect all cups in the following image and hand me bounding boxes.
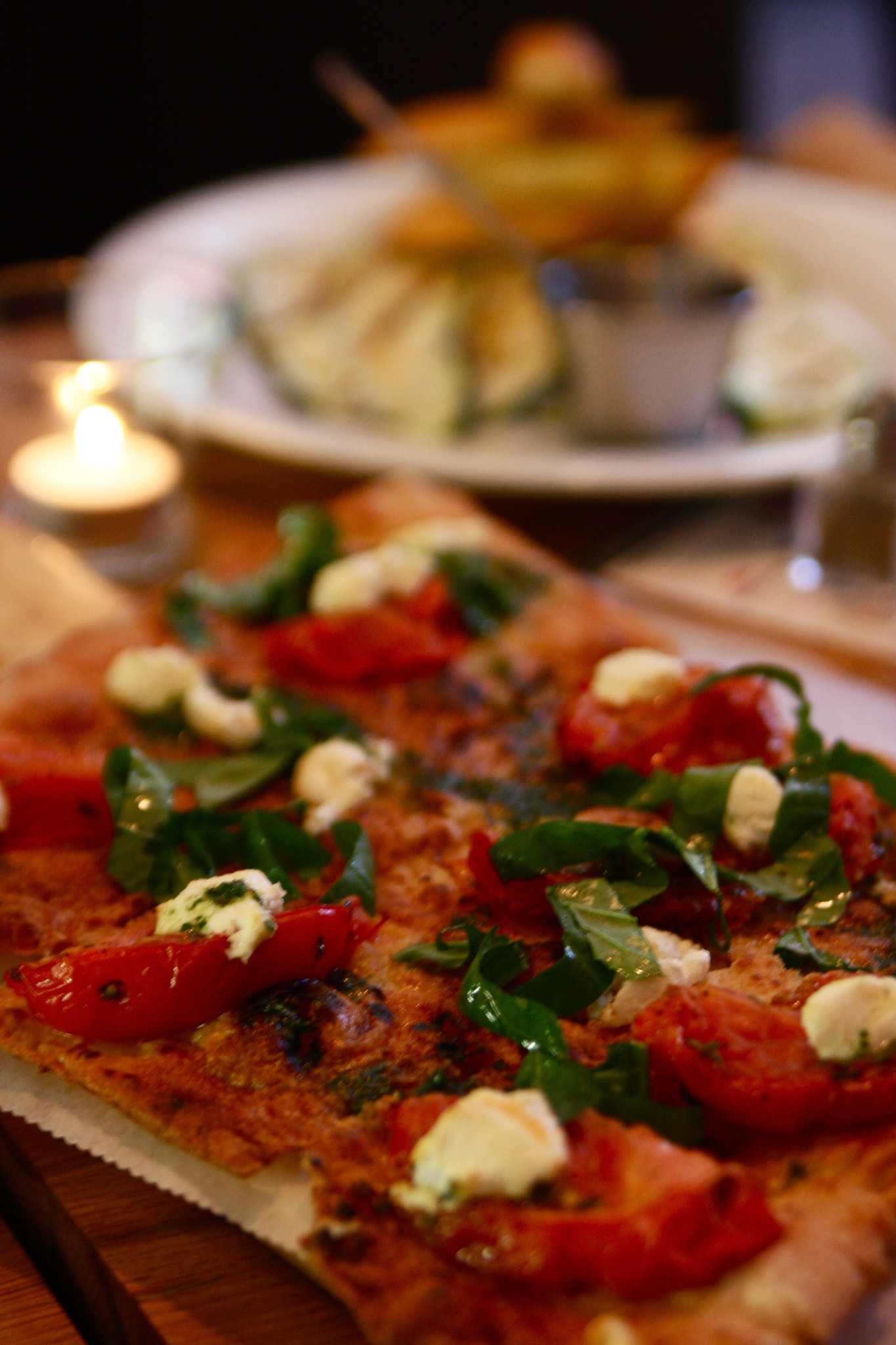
[557,292,735,443]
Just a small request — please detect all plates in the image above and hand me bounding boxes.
[63,153,894,495]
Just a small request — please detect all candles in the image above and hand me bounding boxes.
[5,396,211,592]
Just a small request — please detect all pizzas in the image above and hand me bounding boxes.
[0,471,896,1345]
